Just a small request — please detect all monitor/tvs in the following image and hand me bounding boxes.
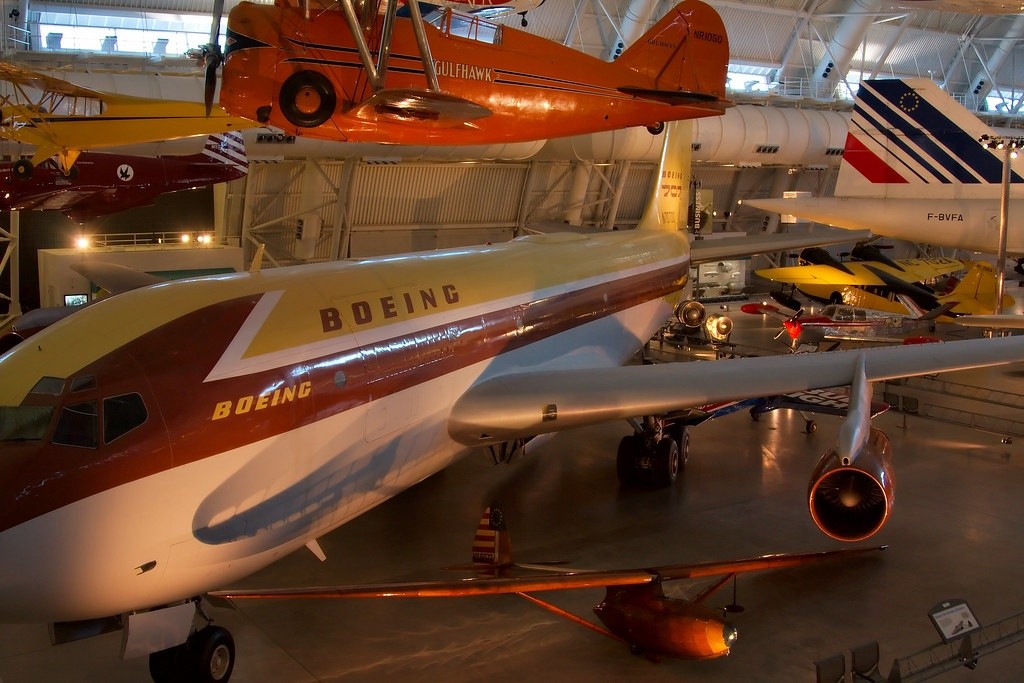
[64,294,88,307]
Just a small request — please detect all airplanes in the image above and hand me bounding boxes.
[0,0,1024,683]
